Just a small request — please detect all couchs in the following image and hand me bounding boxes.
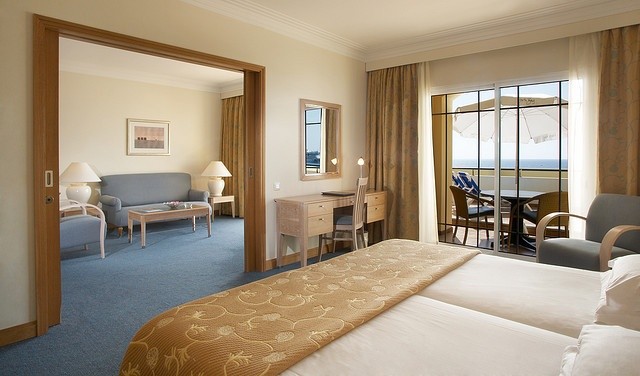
[100,173,209,237]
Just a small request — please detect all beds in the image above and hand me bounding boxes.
[119,239,640,376]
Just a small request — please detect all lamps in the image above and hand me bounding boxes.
[330,157,337,166]
[59,162,102,207]
[358,156,365,178]
[201,161,232,196]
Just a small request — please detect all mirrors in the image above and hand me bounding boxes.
[300,99,341,180]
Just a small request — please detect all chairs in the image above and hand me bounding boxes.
[508,192,568,249]
[457,172,541,206]
[450,186,504,247]
[318,177,369,262]
[536,194,640,271]
[451,171,474,196]
[59,200,107,259]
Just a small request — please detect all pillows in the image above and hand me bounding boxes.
[606,253,640,311]
[573,324,640,376]
[561,346,579,376]
[594,270,640,330]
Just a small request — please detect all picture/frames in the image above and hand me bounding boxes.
[127,118,170,156]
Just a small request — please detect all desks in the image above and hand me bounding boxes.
[208,196,235,222]
[275,190,388,267]
[487,194,542,252]
[59,206,99,217]
